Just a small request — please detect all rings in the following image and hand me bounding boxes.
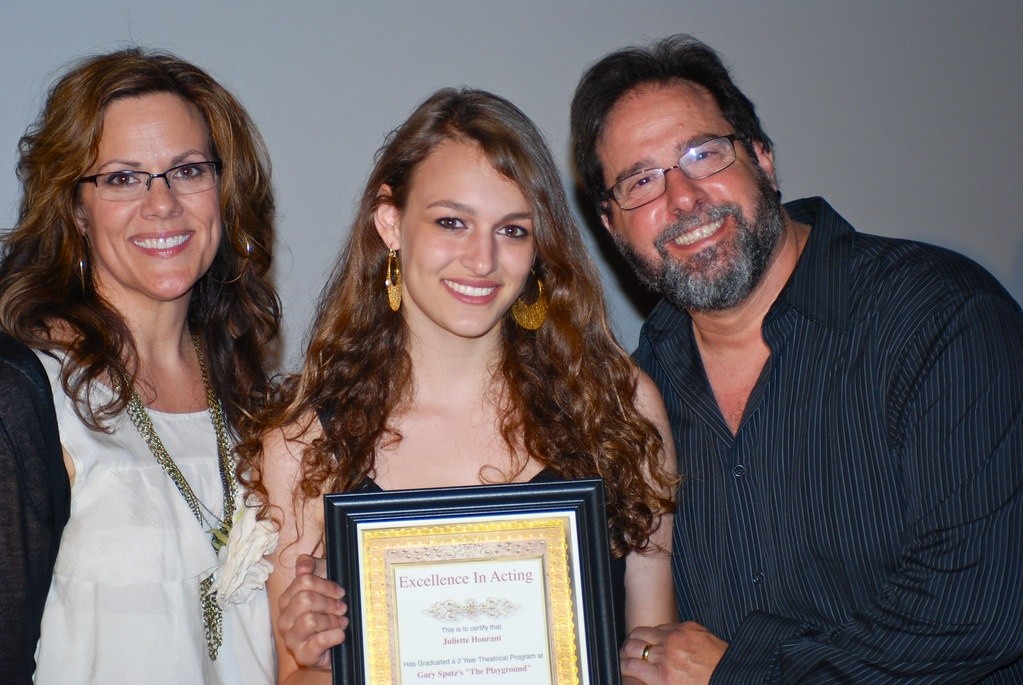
[642,642,652,662]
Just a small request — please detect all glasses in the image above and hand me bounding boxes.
[599,130,748,211]
[75,158,225,196]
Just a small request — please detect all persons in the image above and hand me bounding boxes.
[1,33,1023,685]
[247,85,677,685]
